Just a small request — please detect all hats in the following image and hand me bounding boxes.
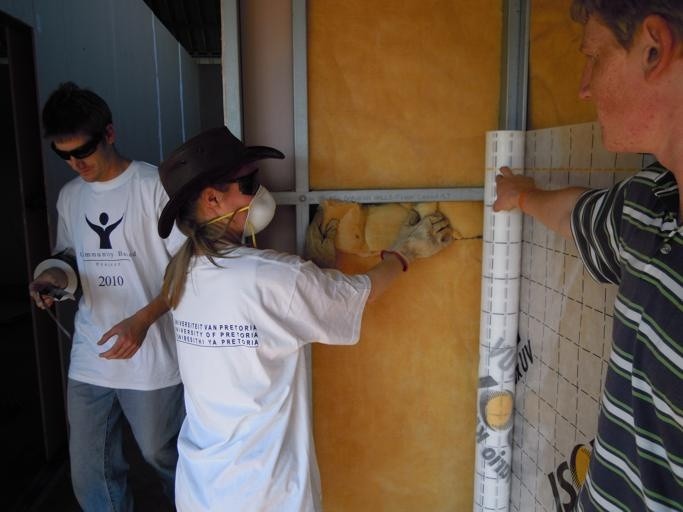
[151,124,285,239]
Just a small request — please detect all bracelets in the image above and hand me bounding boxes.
[519,188,540,214]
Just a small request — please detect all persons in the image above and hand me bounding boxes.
[156,123,453,512]
[26,80,190,512]
[489,1,683,511]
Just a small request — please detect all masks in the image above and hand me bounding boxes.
[241,184,279,243]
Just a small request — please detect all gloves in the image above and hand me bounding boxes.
[379,208,456,274]
[303,206,342,271]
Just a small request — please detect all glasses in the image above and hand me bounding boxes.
[50,135,104,163]
[226,175,259,197]
